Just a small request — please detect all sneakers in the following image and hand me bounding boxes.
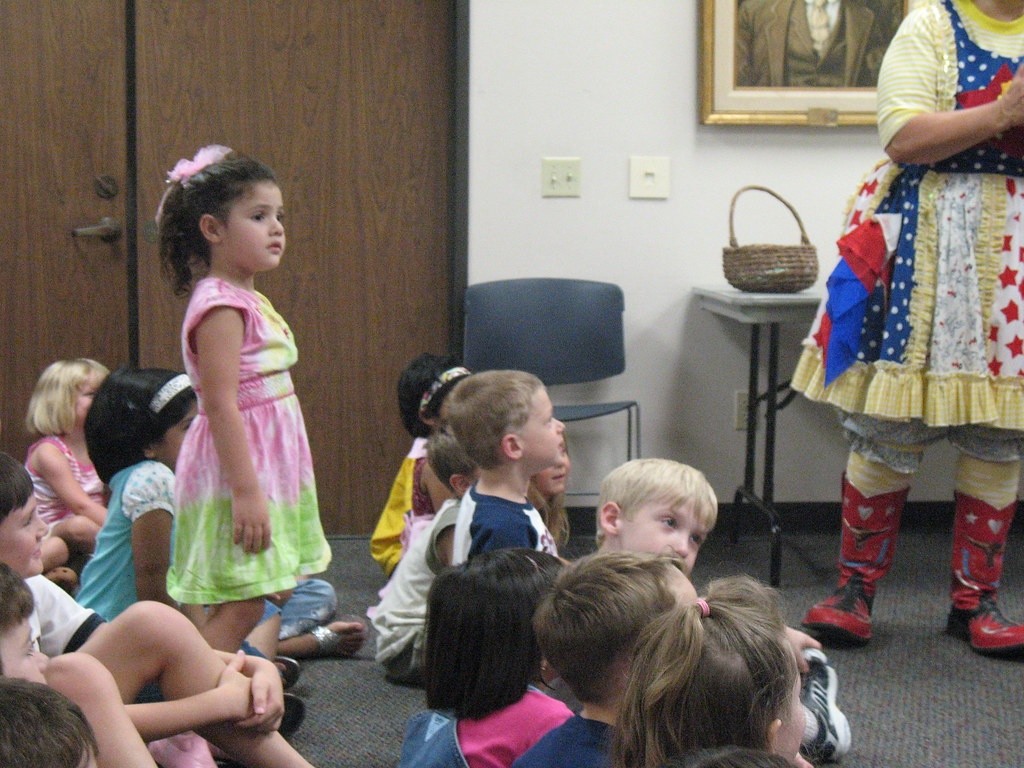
[793,648,853,763]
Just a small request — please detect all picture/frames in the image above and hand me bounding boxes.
[697,0,915,128]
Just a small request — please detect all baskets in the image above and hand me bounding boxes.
[720,184,820,294]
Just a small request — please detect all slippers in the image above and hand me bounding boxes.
[306,613,369,656]
[270,654,306,732]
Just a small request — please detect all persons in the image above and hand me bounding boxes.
[0,148,367,768]
[372,354,853,768]
[733,1,906,87]
[800,0,1023,656]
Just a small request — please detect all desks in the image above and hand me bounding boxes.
[692,284,833,589]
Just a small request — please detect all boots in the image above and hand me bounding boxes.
[945,490,1024,659]
[803,477,910,646]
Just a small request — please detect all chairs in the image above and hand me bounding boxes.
[459,279,641,498]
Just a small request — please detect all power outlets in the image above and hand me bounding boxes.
[734,390,761,431]
